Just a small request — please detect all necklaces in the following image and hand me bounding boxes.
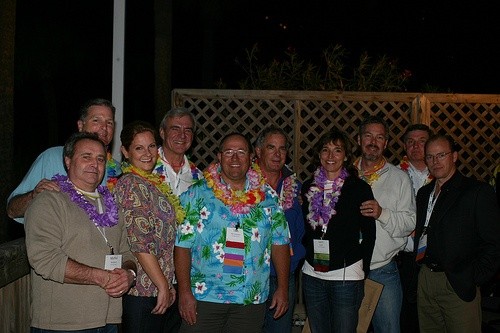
[103,149,120,195]
[354,154,386,185]
[307,167,345,230]
[155,152,200,186]
[205,161,268,213]
[118,159,186,224]
[252,155,298,211]
[400,156,433,187]
[51,173,120,228]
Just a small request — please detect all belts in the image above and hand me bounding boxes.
[423,263,445,273]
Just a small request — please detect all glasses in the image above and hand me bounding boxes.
[425,151,453,161]
[222,148,249,157]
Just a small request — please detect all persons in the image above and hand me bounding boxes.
[415,133,500,333]
[350,115,418,333]
[112,124,178,333]
[23,131,139,333]
[152,105,206,198]
[301,130,377,333]
[4,96,123,225]
[250,126,309,333]
[173,132,293,333]
[395,123,433,332]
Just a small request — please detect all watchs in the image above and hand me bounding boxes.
[127,267,138,290]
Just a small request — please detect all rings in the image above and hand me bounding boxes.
[370,208,374,213]
